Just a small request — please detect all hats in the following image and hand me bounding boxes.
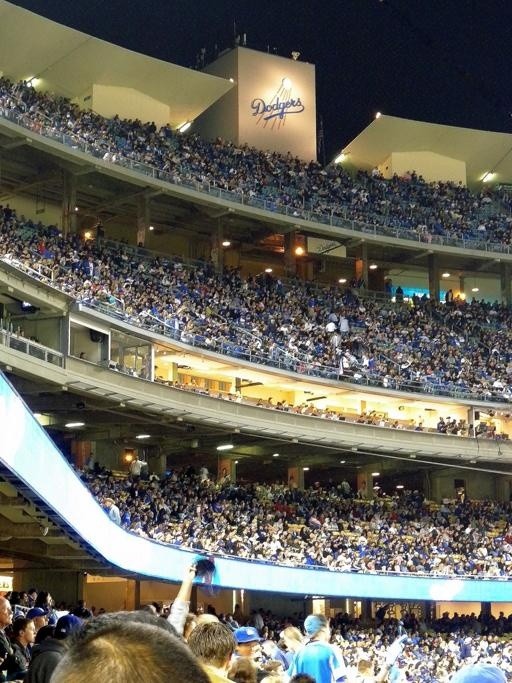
[57,614,81,633]
[233,627,265,643]
[303,614,325,639]
[26,607,49,619]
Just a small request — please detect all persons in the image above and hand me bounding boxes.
[1,80,512,682]
